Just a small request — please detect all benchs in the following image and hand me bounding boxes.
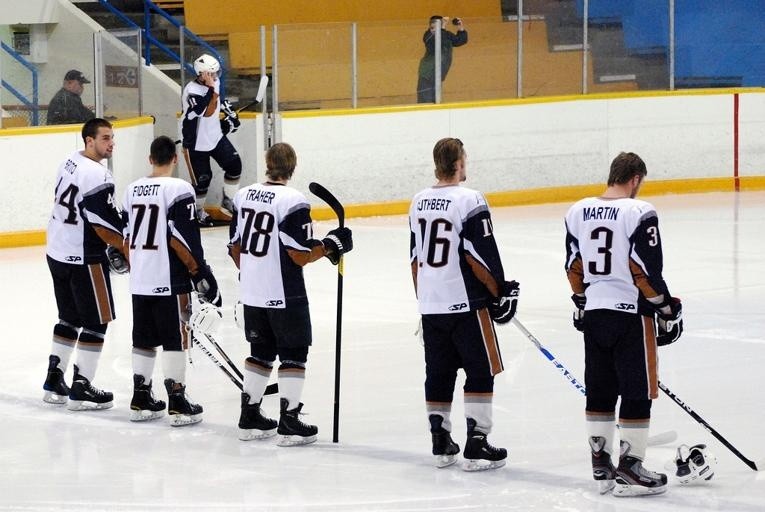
[153,0,761,111]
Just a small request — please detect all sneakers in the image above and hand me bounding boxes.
[195,209,213,226]
[592,451,617,480]
[615,454,670,487]
[221,195,234,215]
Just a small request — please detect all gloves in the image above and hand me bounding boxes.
[490,280,520,323]
[192,267,221,307]
[322,228,353,266]
[220,119,230,134]
[656,298,683,346]
[572,293,585,334]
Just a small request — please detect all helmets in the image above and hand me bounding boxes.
[104,245,128,274]
[188,303,220,333]
[235,299,244,331]
[671,444,716,486]
[194,55,221,83]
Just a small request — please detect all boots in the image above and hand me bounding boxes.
[277,399,317,436]
[429,413,460,454]
[70,364,113,403]
[43,368,70,396]
[463,418,507,459]
[239,392,277,430]
[131,374,166,411]
[164,379,202,415]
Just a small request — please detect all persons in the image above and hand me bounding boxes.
[180,54,241,227]
[228,143,352,438]
[43,119,124,404]
[417,16,467,104]
[122,136,221,416]
[409,138,520,461]
[48,70,95,123]
[565,152,683,488]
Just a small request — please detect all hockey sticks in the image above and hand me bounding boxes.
[175,75,270,145]
[309,182,354,442]
[657,378,765,472]
[192,331,278,396]
[511,317,676,447]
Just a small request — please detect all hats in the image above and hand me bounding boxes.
[64,70,91,84]
